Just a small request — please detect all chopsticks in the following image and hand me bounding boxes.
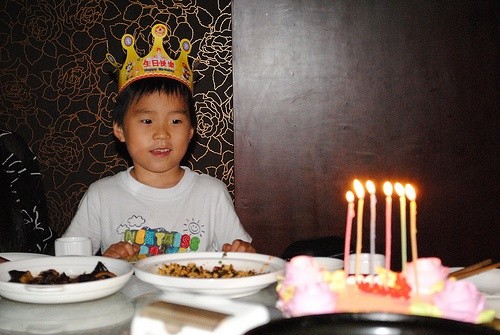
[448,259,500,281]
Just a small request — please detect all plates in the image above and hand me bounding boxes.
[0,293,134,333]
[243,311,500,335]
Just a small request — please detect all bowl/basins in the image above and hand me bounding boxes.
[0,255,134,304]
[445,265,500,321]
[134,251,289,299]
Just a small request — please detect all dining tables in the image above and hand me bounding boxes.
[0,274,281,335]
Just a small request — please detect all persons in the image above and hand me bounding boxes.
[61,24,256,261]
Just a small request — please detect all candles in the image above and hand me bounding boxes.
[353,179,365,253]
[384,181,392,270]
[406,183,417,261]
[394,181,408,266]
[343,190,355,270]
[366,180,376,254]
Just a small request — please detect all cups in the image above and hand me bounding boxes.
[349,254,385,274]
[55,237,93,257]
[312,256,345,270]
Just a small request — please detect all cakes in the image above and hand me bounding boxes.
[276,255,496,326]
